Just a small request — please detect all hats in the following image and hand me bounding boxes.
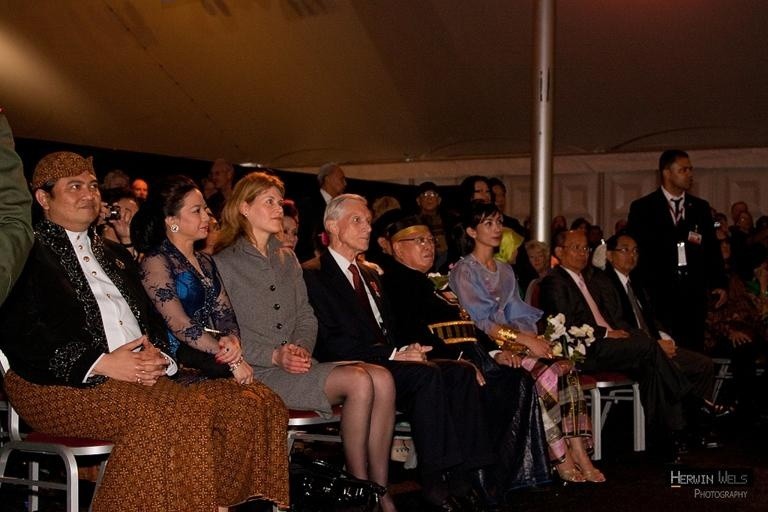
[418,182,440,194]
[31,151,96,189]
[386,216,425,237]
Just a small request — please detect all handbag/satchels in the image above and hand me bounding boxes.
[296,457,386,512]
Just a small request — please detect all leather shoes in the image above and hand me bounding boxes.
[463,486,492,512]
[698,429,722,449]
[697,399,738,419]
[428,497,463,512]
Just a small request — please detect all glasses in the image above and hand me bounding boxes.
[396,236,435,243]
[610,247,639,254]
[474,188,490,194]
[561,244,593,252]
[718,237,729,245]
[420,192,438,198]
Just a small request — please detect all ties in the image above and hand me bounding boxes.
[579,282,613,331]
[626,280,650,338]
[669,197,683,224]
[348,263,381,336]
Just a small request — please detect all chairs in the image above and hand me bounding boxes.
[523,278,646,462]
[0,350,114,512]
[287,406,343,461]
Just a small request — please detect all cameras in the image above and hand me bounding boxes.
[104,205,121,222]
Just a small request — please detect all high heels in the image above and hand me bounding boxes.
[551,453,584,482]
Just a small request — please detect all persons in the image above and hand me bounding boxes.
[301,162,347,251]
[92,188,141,245]
[380,212,532,512]
[0,106,35,310]
[130,174,289,512]
[448,200,608,481]
[618,148,729,355]
[537,229,739,484]
[413,181,449,257]
[0,152,213,511]
[553,211,627,242]
[280,198,300,251]
[453,175,496,209]
[705,199,768,455]
[489,177,537,293]
[213,172,397,512]
[130,178,149,201]
[303,191,498,511]
[523,240,552,280]
[202,157,234,227]
[590,231,716,398]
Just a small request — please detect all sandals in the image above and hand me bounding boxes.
[568,449,606,482]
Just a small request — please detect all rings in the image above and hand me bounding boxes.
[511,354,517,359]
[134,377,143,386]
[222,347,230,354]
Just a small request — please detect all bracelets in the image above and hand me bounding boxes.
[228,356,245,374]
[497,321,521,344]
